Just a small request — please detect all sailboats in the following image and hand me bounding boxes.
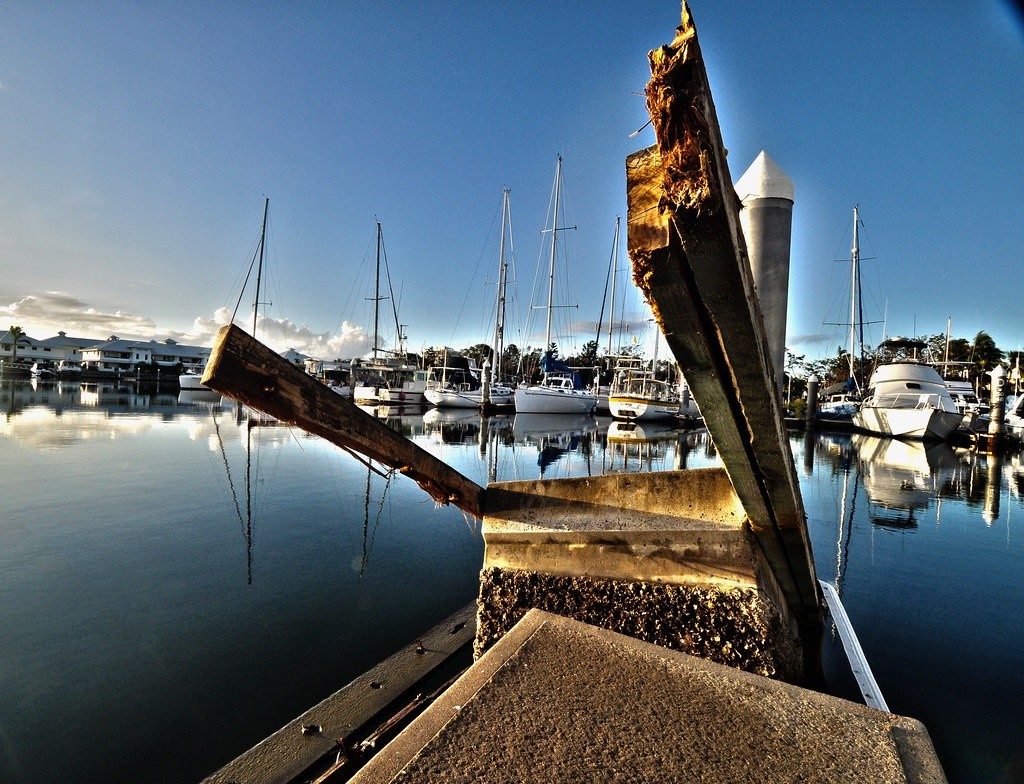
[593,214,698,418]
[419,408,513,455]
[424,186,521,407]
[508,418,609,481]
[513,154,609,412]
[353,403,426,584]
[178,196,425,435]
[178,388,296,585]
[604,422,708,474]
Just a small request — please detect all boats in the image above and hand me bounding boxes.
[818,394,865,419]
[850,430,966,535]
[851,336,966,435]
